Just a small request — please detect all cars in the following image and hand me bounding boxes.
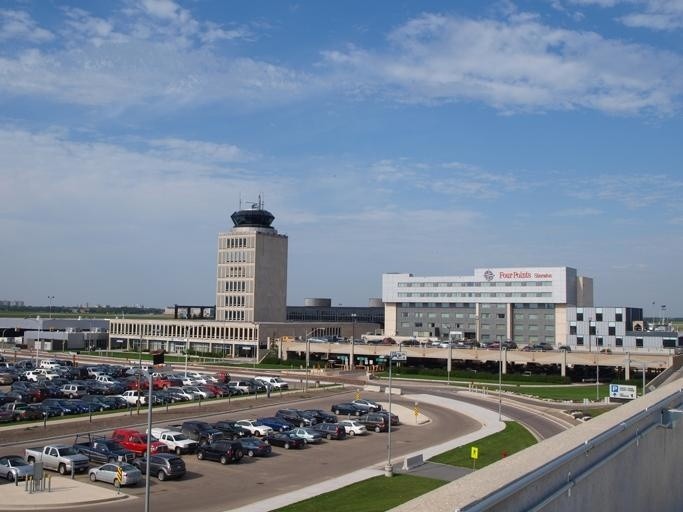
[0,455,35,481]
[88,461,141,487]
[277,335,295,343]
[331,398,399,436]
[180,408,345,465]
[399,339,518,351]
[0,353,289,423]
[521,342,554,352]
[308,334,346,344]
[133,452,186,481]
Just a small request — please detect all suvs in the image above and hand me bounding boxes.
[73,433,135,465]
[113,426,198,460]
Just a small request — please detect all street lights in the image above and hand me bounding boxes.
[306,326,326,368]
[24,317,55,369]
[631,360,667,396]
[125,366,172,512]
[172,324,202,378]
[48,295,55,318]
[381,354,402,477]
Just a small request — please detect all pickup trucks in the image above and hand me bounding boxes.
[24,444,89,475]
